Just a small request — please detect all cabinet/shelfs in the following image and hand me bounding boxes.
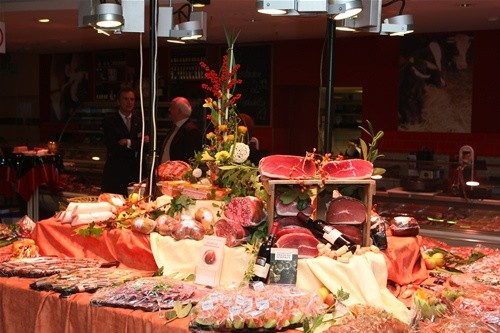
[57,108,211,176]
[90,45,214,101]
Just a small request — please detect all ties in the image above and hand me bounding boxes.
[158,125,177,166]
[126,117,131,131]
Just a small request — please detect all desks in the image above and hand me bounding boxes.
[0,216,500,333]
[0,152,65,223]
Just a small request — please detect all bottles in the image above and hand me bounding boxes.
[95,86,119,101]
[169,56,206,79]
[248,221,281,290]
[297,212,355,251]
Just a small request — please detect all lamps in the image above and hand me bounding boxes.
[78,0,208,201]
[256,0,415,154]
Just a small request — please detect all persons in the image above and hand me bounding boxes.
[101,86,148,197]
[64,53,87,107]
[447,34,471,81]
[233,114,267,167]
[142,97,204,164]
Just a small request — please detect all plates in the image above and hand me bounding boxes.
[12,147,46,156]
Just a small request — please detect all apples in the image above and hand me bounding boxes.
[422,251,444,269]
[318,287,336,308]
[12,239,35,250]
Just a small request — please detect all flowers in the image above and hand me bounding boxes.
[0,219,23,240]
[413,286,459,328]
[429,248,485,266]
[302,286,350,333]
[183,25,271,282]
[281,119,386,221]
